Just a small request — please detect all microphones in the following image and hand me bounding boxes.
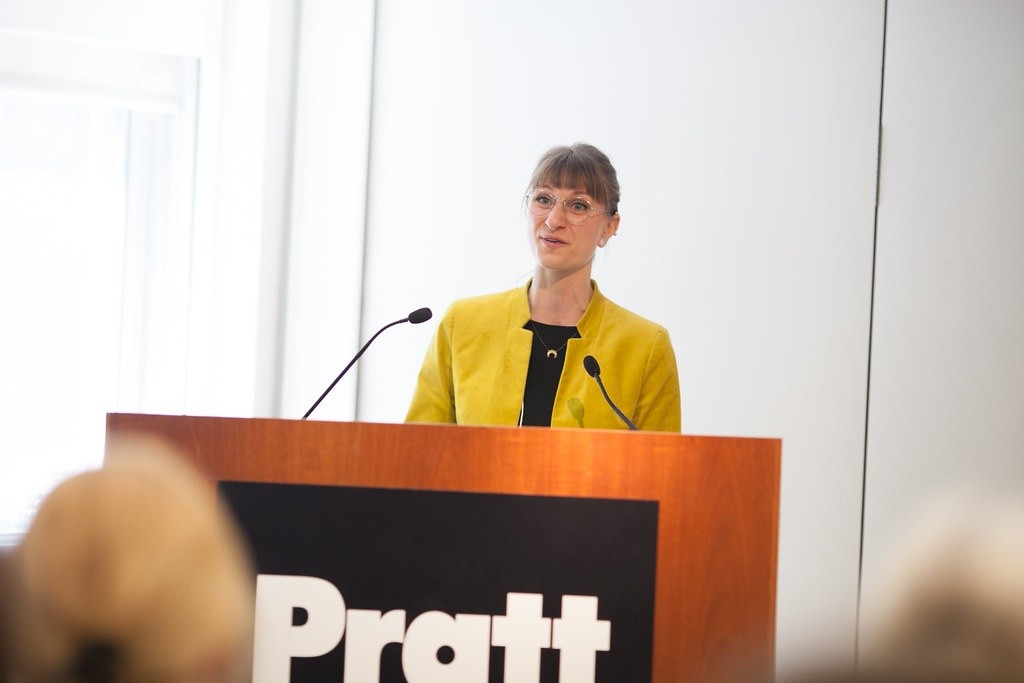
[582,354,638,431]
[299,308,433,422]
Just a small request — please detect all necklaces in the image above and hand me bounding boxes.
[531,320,577,358]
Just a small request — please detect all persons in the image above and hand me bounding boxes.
[404,142,682,431]
[1,465,258,683]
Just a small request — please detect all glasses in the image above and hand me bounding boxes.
[526,190,611,225]
